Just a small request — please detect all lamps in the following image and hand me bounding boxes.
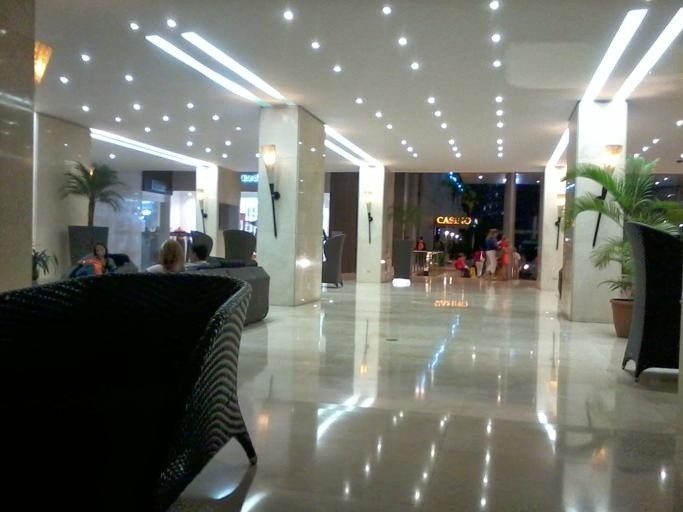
[363,192,374,245]
[258,143,281,238]
[193,187,208,235]
[552,193,565,251]
[588,142,623,249]
[33,38,52,91]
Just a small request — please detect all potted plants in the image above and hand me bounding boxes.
[30,246,59,282]
[553,156,682,336]
[385,199,425,280]
[60,158,124,263]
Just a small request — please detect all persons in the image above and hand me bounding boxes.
[146,233,213,272]
[160,239,184,273]
[454,229,524,279]
[81,242,117,278]
[416,236,427,267]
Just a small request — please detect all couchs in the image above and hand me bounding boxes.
[62,250,137,282]
[186,256,270,329]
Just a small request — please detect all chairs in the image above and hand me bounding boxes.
[191,230,213,255]
[319,233,346,288]
[0,272,256,511]
[617,218,682,383]
[222,229,256,262]
